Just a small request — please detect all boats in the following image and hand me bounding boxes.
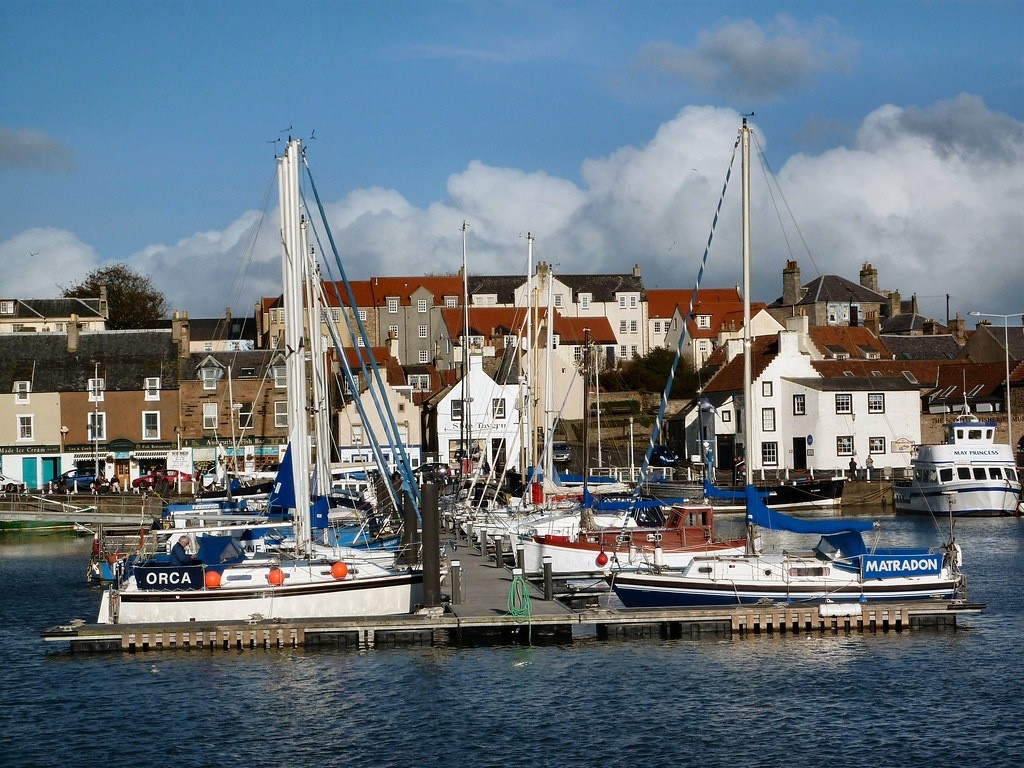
[887,415,1023,516]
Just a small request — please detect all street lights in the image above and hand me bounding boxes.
[964,311,1014,454]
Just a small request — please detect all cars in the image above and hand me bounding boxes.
[0,475,27,494]
[589,401,607,416]
[553,441,572,463]
[132,469,192,487]
[337,468,376,483]
[49,467,104,492]
[648,405,661,415]
[649,444,679,467]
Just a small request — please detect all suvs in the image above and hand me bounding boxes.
[411,462,452,481]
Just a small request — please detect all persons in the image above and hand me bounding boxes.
[56,473,121,494]
[169,536,196,567]
[849,455,875,481]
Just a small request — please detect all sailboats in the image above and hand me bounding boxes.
[95,109,969,629]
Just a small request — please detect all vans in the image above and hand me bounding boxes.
[609,400,640,414]
